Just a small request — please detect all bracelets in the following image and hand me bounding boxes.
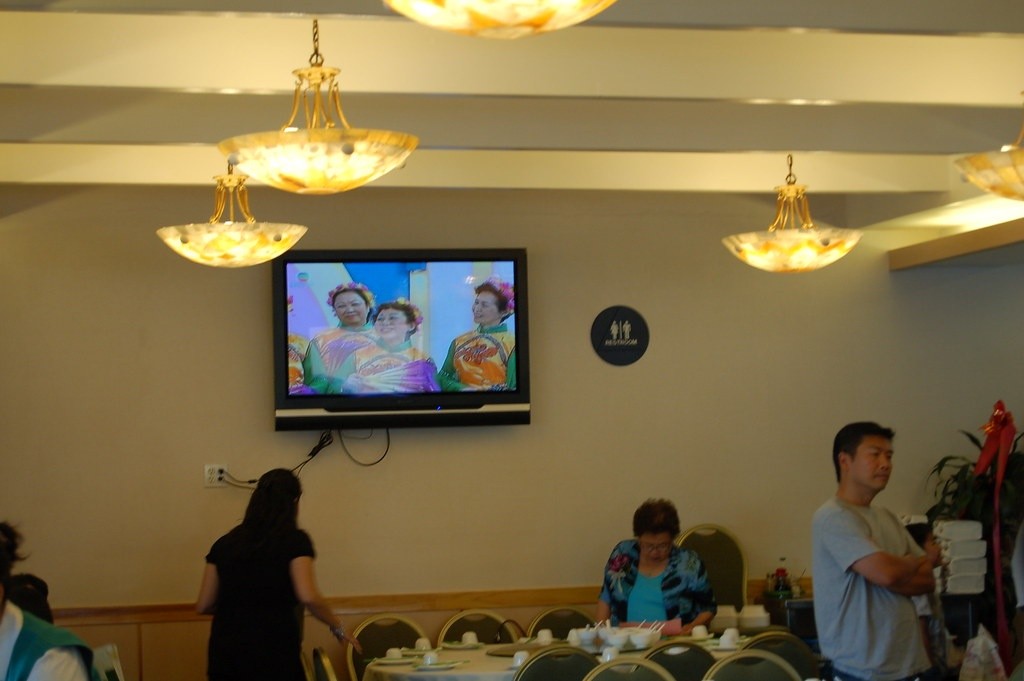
[331,623,347,637]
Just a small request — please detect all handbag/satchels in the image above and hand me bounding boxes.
[959,623,1006,681]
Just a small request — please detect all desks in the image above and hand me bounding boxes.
[758,595,820,652]
[361,635,740,681]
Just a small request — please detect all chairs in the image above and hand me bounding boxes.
[677,524,748,611]
[304,605,823,681]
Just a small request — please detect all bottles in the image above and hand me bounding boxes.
[792,581,800,598]
[776,558,787,591]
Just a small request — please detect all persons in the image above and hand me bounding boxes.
[436,274,516,391]
[1011,518,1024,660]
[327,296,442,394]
[312,283,377,394]
[195,467,362,681]
[812,421,936,681]
[287,291,317,394]
[594,496,718,636]
[0,520,102,681]
[905,522,951,681]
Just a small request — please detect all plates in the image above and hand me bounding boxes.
[442,643,484,650]
[415,662,463,670]
[739,604,770,627]
[378,657,416,665]
[710,646,738,651]
[711,605,738,630]
[693,634,713,641]
[509,665,521,670]
[402,646,442,656]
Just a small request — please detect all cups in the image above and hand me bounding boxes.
[719,628,738,646]
[512,651,529,665]
[424,652,437,665]
[415,637,431,651]
[462,631,477,644]
[602,647,619,663]
[539,629,553,644]
[385,648,402,660]
[693,625,707,639]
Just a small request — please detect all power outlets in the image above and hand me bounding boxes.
[204,464,231,489]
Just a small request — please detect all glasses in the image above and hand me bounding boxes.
[639,539,672,551]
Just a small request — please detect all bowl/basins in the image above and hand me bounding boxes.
[577,628,596,643]
[606,633,628,648]
[625,627,661,649]
[599,627,618,640]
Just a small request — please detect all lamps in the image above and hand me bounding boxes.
[217,16,420,197]
[721,152,864,272]
[155,157,308,267]
[386,1,617,39]
[949,115,1024,202]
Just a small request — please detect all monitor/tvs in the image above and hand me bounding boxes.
[273,248,531,431]
